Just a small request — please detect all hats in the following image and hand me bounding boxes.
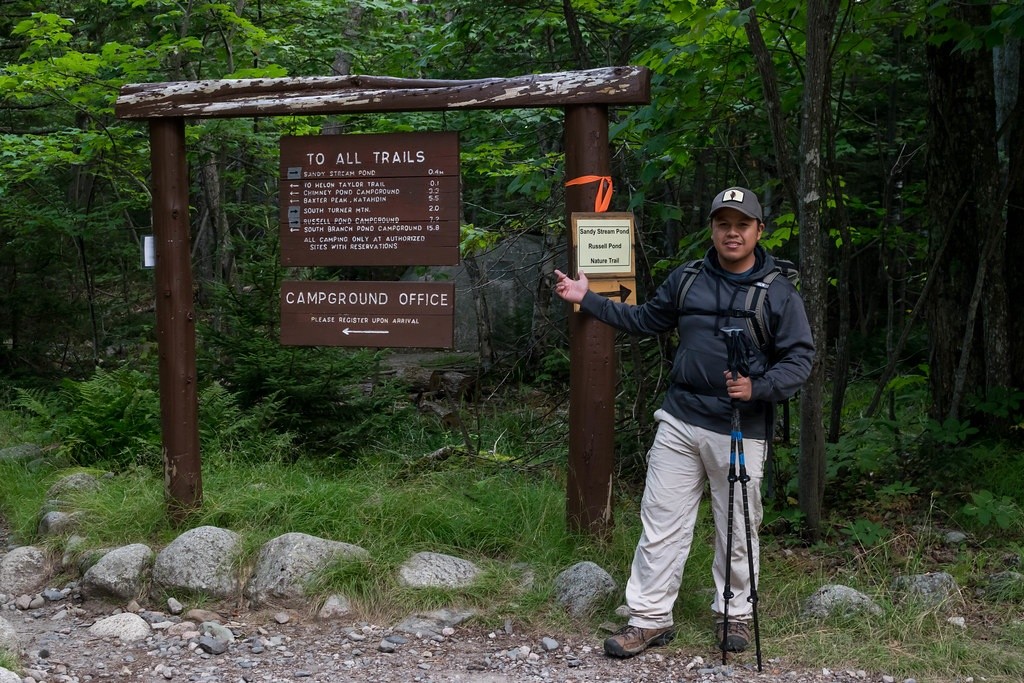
[708,187,763,224]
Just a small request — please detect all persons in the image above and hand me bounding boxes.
[553,187,815,658]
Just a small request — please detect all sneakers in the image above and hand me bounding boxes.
[716,619,751,652]
[604,622,674,658]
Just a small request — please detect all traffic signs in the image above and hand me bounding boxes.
[279,280,454,349]
[280,131,462,267]
